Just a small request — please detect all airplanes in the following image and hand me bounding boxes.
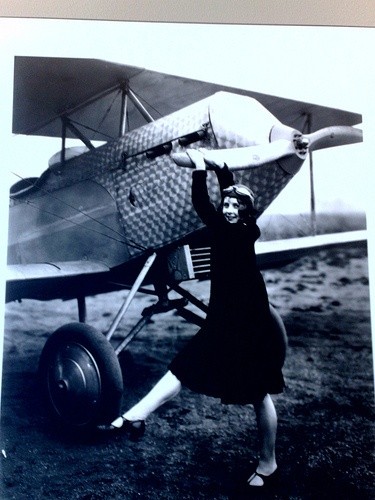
[1,54,364,435]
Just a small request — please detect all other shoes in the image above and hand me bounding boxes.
[231,464,286,500]
[98,415,145,442]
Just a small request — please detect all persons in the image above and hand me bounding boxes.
[88,148,284,500]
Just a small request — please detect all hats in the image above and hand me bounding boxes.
[222,183,255,210]
